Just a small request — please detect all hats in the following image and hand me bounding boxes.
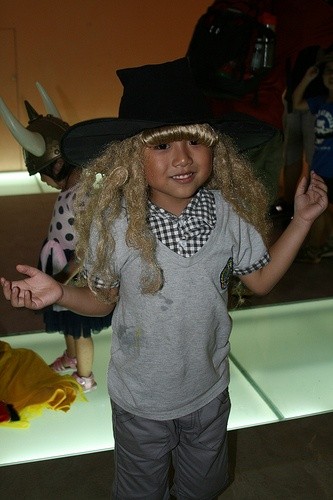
[311,38,333,76]
[115,57,218,141]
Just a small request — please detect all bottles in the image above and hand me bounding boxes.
[255,12,277,73]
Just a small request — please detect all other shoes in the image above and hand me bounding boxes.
[318,246,333,257]
[294,251,321,264]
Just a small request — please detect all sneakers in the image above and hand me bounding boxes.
[69,373,97,394]
[52,353,78,376]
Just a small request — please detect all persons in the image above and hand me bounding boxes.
[0,87,329,500]
[21,115,113,395]
[270,45,333,263]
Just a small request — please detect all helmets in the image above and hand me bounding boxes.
[0,79,73,175]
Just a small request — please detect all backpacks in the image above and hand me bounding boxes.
[185,5,272,101]
